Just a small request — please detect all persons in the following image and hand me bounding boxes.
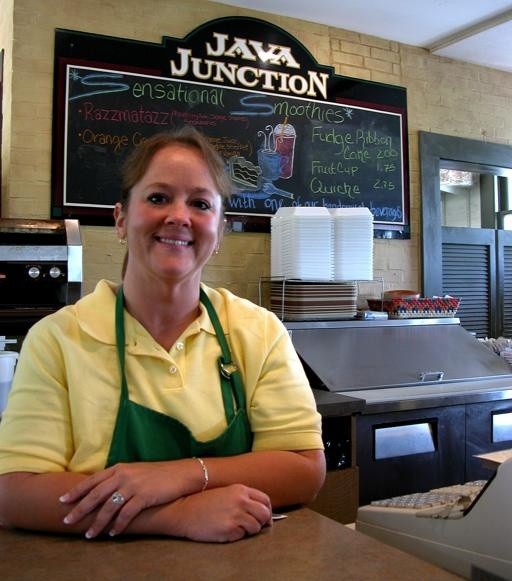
[0,126,327,542]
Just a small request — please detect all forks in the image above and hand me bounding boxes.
[262,183,294,200]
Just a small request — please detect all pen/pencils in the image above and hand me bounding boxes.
[273,503,307,514]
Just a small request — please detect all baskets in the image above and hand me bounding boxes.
[367,298,462,318]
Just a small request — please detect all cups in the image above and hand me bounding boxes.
[271,132,296,179]
[0,349,20,420]
[257,148,282,181]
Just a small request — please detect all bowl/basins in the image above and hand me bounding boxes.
[384,290,420,300]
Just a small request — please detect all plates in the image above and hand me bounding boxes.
[269,205,375,283]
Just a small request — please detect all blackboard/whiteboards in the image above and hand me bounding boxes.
[64,64,405,225]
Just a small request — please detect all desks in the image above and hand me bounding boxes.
[0,504,468,581]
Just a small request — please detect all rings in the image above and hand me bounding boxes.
[111,492,127,506]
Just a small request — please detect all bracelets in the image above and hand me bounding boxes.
[192,455,210,491]
